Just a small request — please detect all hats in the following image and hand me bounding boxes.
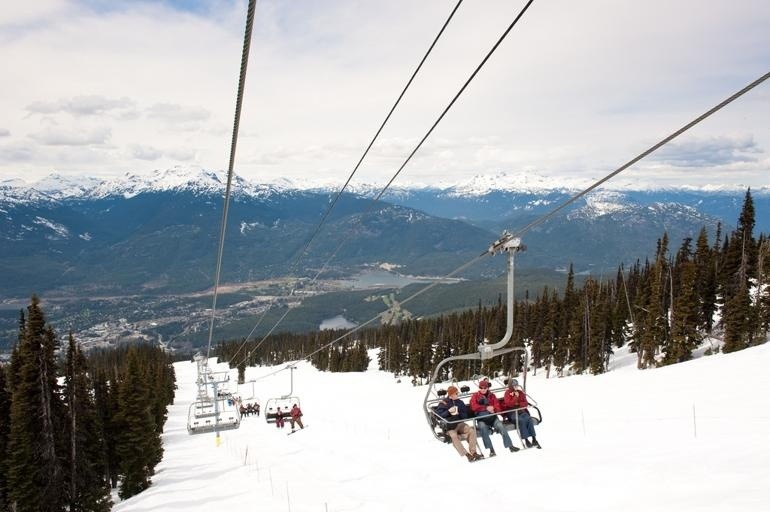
[511,379,518,385]
[448,386,458,396]
[479,381,487,387]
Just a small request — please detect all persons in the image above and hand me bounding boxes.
[218,391,307,435]
[469,380,520,456]
[501,378,542,450]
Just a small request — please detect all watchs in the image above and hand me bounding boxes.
[437,385,484,462]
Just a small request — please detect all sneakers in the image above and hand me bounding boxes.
[468,454,482,461]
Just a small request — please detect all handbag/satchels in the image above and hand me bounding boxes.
[475,398,492,420]
